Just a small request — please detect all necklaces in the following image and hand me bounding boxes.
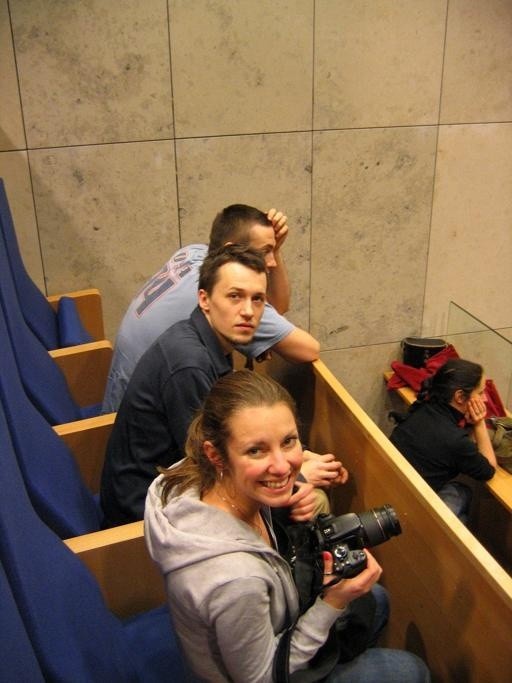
[218,491,264,532]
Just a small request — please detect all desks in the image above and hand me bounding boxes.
[383,371,512,515]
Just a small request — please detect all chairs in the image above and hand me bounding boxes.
[0,178,114,406]
[0,560,47,683]
[1,302,168,630]
[0,395,204,683]
[0,231,119,497]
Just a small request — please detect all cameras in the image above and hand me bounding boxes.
[300,502,403,579]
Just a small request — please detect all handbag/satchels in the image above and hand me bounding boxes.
[402,336,447,369]
[486,415,511,468]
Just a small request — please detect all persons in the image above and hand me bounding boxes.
[390,359,497,525]
[100,203,321,416]
[101,243,349,523]
[144,369,390,683]
